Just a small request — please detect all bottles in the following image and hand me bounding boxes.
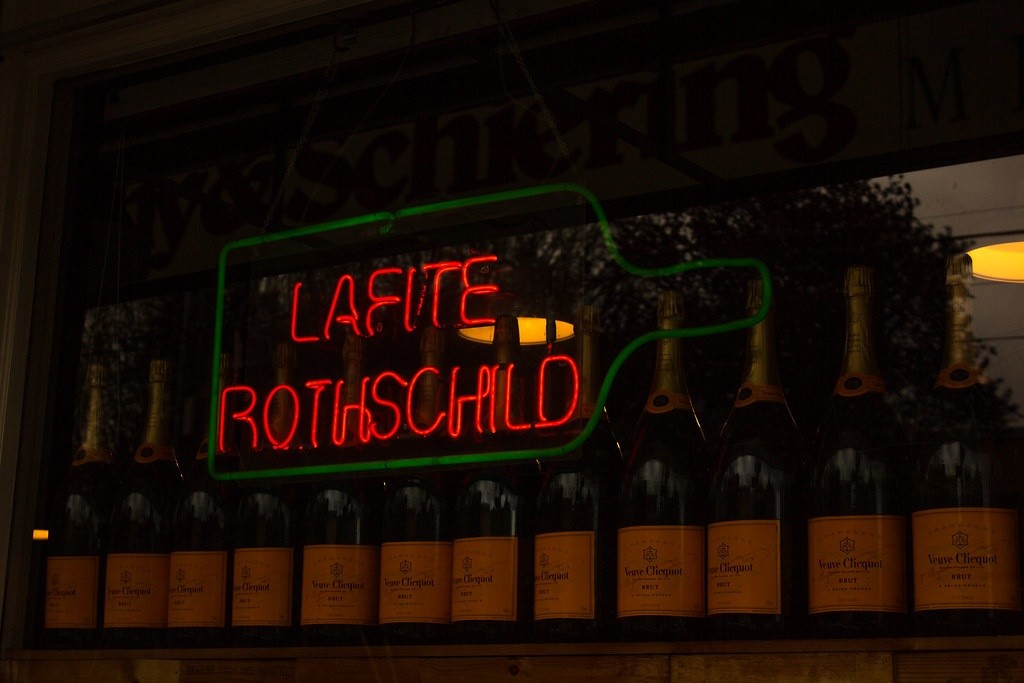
[804,270,904,634]
[298,336,379,638]
[611,286,704,636]
[101,361,187,644]
[909,254,1018,634]
[707,282,799,637]
[166,351,231,643]
[528,307,609,638]
[42,366,122,649]
[229,344,300,641]
[379,326,449,637]
[450,316,528,638]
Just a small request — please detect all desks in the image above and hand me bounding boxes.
[0,635,1024,683]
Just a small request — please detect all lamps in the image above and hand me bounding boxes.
[963,234,1024,283]
[454,312,575,345]
